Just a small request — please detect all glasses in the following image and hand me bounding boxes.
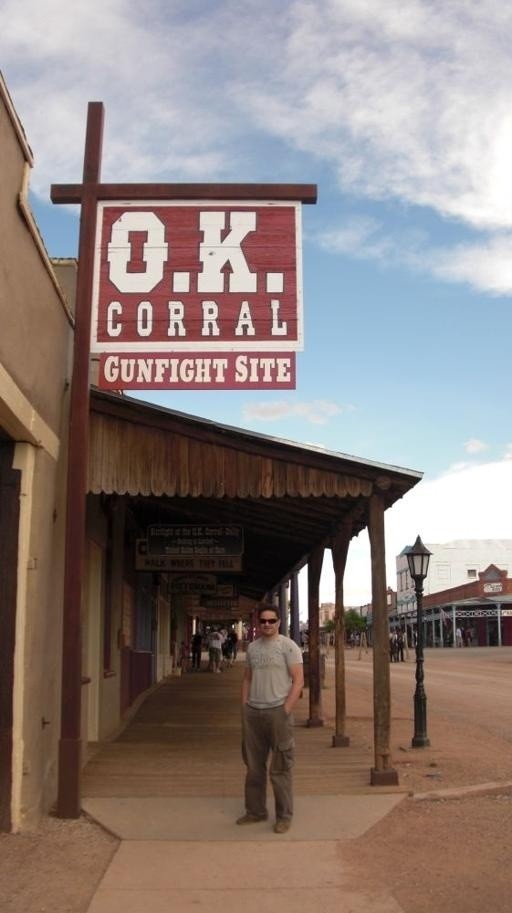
[259,618,280,624]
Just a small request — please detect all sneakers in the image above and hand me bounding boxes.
[235,809,268,824]
[273,814,293,834]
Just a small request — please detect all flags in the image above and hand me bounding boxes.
[441,609,450,628]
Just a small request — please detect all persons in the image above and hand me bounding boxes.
[186,617,238,674]
[235,602,305,835]
[299,625,472,663]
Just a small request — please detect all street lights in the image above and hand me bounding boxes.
[404,535,433,747]
